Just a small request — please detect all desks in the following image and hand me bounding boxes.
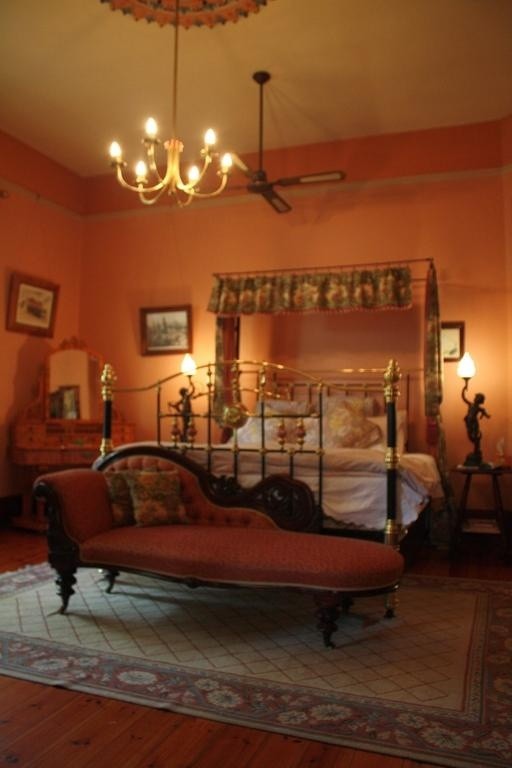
[446,465,512,563]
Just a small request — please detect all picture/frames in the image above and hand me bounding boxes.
[438,318,466,364]
[3,269,64,340]
[135,303,198,358]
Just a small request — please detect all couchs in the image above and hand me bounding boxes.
[30,445,406,651]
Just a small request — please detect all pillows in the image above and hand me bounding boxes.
[253,397,312,417]
[104,469,138,529]
[317,409,408,452]
[123,468,189,528]
[317,391,382,415]
[227,414,322,448]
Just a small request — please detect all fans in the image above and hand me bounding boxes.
[209,70,347,214]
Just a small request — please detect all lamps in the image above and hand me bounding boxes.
[456,351,491,465]
[97,0,269,211]
[172,351,204,446]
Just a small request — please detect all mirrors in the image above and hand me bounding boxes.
[41,345,96,427]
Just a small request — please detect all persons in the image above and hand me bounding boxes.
[460,384,492,450]
[166,374,198,438]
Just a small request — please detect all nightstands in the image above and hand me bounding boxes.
[11,424,137,531]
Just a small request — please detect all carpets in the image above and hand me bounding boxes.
[1,554,510,768]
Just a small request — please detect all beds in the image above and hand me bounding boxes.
[97,355,448,578]
